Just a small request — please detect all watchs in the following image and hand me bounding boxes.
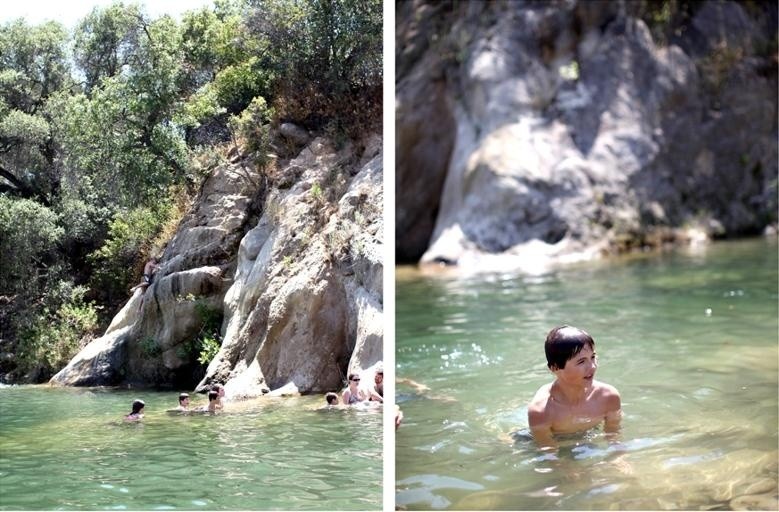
[342,373,367,408]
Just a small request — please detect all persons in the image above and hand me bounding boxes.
[317,392,340,412]
[368,367,383,402]
[503,324,632,483]
[396,377,458,407]
[166,392,205,418]
[395,404,403,429]
[207,390,222,415]
[109,398,161,431]
[211,383,226,408]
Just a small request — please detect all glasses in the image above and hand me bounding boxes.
[353,379,360,381]
